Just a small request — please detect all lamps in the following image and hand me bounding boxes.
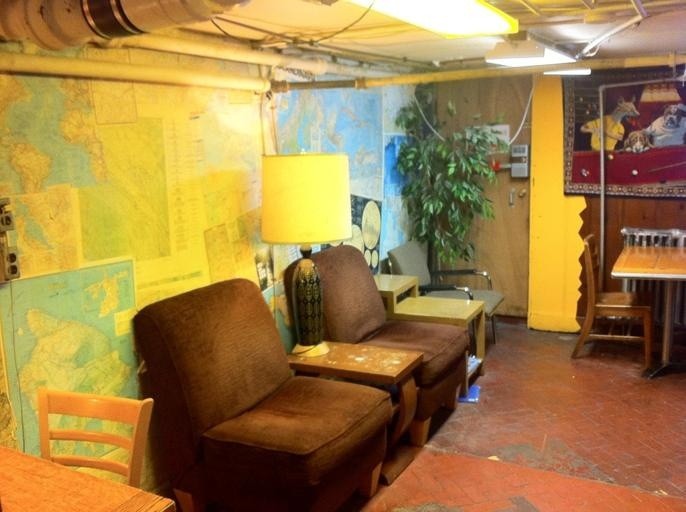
[485,31,592,76]
[260,153,352,359]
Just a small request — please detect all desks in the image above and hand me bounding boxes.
[1,445,177,512]
[610,246,686,380]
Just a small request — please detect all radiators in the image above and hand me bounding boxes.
[618,226,686,336]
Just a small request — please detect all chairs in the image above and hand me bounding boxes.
[387,241,504,345]
[570,233,656,370]
[36,388,155,488]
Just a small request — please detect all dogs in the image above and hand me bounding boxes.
[623,130,655,153]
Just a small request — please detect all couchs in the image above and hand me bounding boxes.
[132,278,394,512]
[283,246,469,447]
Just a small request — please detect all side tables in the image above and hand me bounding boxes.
[373,273,486,398]
[287,342,423,487]
